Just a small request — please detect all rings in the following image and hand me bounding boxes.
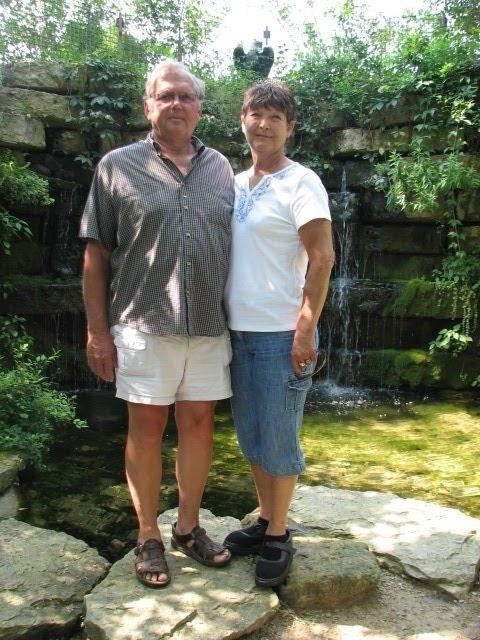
[299,362,306,367]
[306,360,312,364]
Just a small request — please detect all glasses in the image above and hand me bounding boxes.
[158,92,198,102]
[288,350,327,384]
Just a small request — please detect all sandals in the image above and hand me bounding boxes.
[134,539,171,587]
[171,521,231,567]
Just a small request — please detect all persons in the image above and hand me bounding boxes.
[223,80,335,588]
[78,59,234,588]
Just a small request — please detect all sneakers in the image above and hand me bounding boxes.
[224,519,293,586]
